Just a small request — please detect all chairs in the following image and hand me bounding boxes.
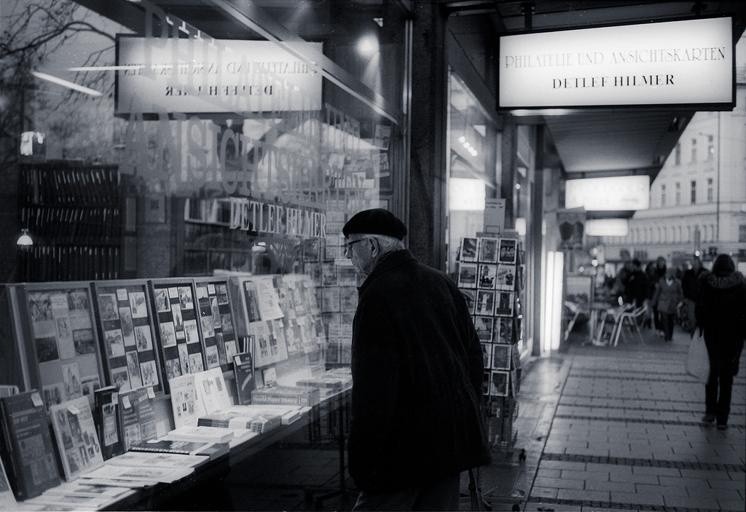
[564,299,648,348]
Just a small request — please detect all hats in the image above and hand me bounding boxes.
[342,208,407,241]
[712,253,735,277]
[667,267,677,277]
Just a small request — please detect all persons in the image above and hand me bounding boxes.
[596,247,713,346]
[342,206,494,511]
[695,252,746,429]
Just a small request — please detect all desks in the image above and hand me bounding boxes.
[0,366,352,512]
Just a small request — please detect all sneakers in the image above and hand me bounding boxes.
[701,414,728,430]
[655,329,672,341]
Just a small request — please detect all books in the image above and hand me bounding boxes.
[454,237,526,398]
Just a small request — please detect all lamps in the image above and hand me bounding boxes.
[16,227,34,246]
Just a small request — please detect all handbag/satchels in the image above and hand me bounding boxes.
[685,333,711,383]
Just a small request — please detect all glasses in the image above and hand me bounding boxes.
[342,236,367,254]
